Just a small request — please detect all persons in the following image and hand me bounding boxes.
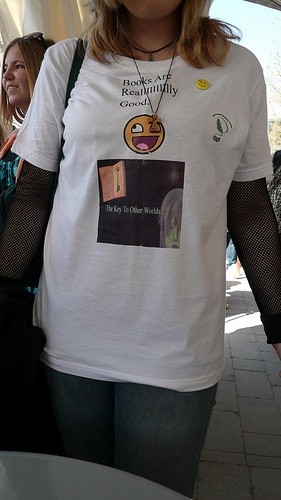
[225,151,281,309]
[0,0,281,498]
[0,32,56,294]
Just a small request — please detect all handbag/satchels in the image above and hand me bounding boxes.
[0,285,58,453]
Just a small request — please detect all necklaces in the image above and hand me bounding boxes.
[120,31,180,129]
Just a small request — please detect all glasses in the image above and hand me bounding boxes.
[22,32,49,52]
